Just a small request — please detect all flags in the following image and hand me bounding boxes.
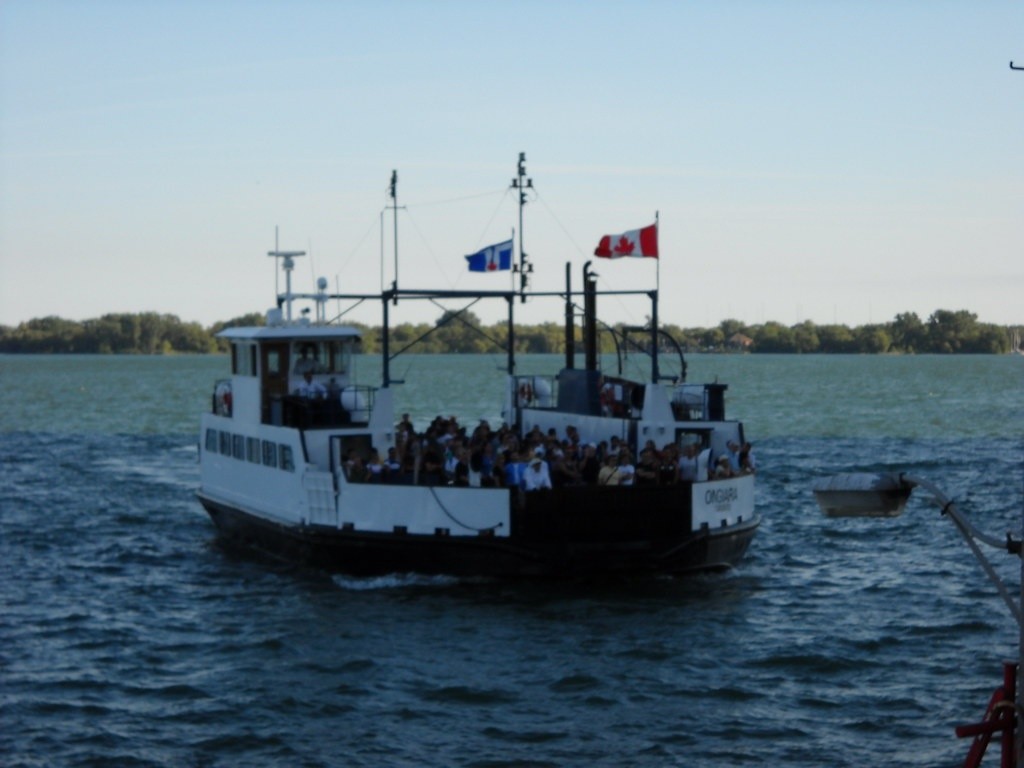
[594,221,658,260]
[464,239,512,273]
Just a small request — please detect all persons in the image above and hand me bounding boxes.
[345,411,755,490]
[294,373,328,399]
[295,346,317,374]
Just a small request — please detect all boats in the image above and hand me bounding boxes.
[191,149,758,586]
